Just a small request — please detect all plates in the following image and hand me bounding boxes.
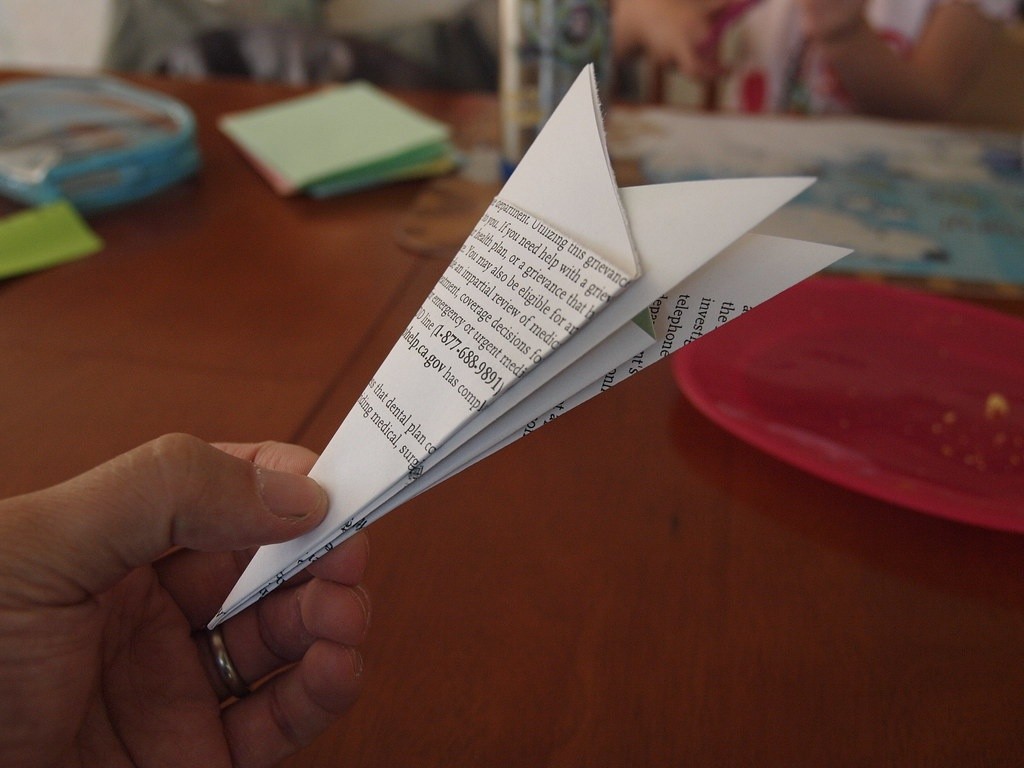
[668,274,1024,536]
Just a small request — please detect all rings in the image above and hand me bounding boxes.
[207,623,252,699]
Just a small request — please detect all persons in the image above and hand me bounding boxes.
[610,0,1024,139]
[0,431,375,768]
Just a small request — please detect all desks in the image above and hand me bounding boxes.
[0,70,1023,768]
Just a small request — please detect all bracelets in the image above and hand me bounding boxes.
[814,17,870,43]
